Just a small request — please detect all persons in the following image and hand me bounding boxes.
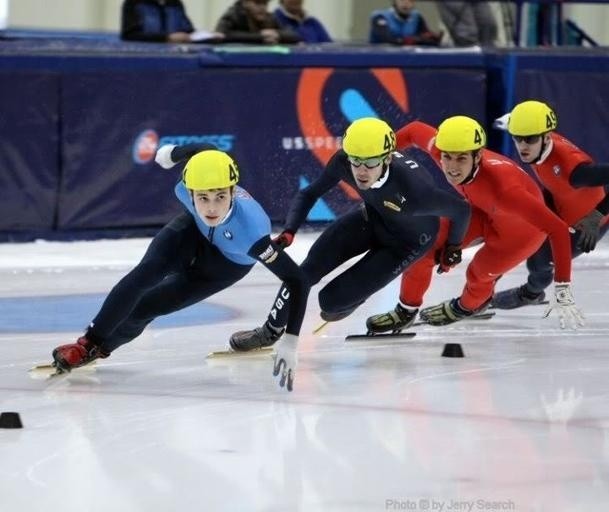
[491,100,609,310]
[52,142,310,392]
[366,115,585,332]
[368,0,439,46]
[120,0,193,43]
[276,0,332,43]
[229,117,472,352]
[215,0,302,45]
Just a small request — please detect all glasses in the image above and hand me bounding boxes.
[514,136,541,144]
[349,155,387,169]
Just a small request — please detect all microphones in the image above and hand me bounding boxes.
[567,18,601,47]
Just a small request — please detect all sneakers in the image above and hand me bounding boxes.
[53,337,104,369]
[367,284,545,332]
[229,320,285,350]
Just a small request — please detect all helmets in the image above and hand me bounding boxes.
[343,118,395,157]
[508,100,557,136]
[185,150,239,191]
[435,116,486,152]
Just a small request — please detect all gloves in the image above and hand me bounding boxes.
[436,243,461,274]
[272,232,294,250]
[273,333,298,391]
[573,209,604,252]
[542,281,585,330]
